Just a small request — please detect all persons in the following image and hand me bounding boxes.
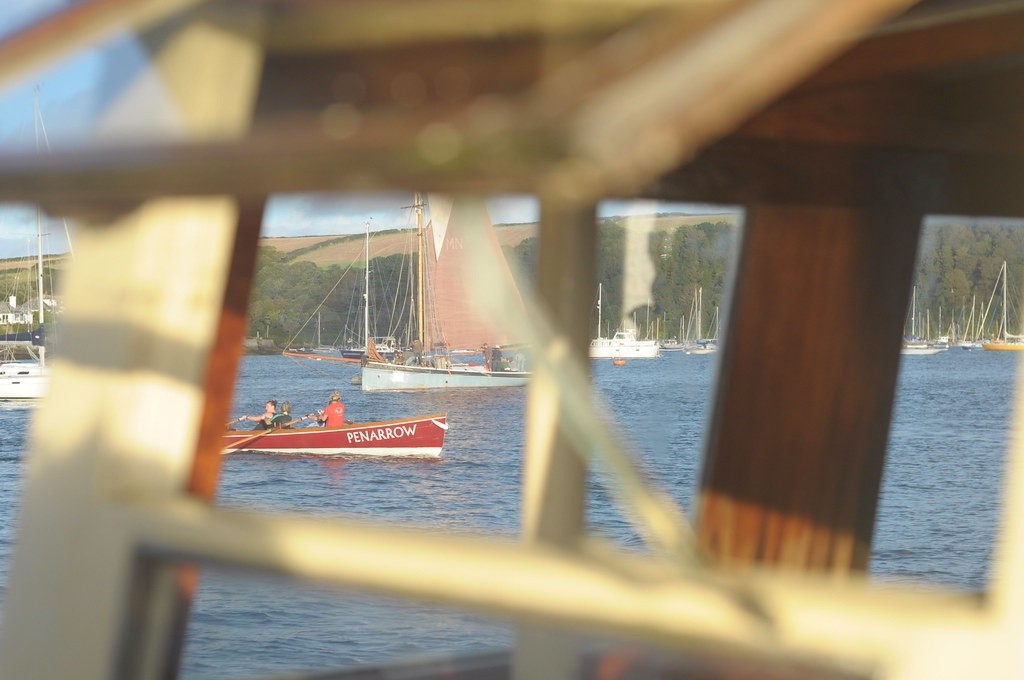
[480,344,501,372]
[412,338,424,367]
[307,391,344,427]
[238,400,292,430]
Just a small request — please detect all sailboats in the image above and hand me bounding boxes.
[899,261,1024,354]
[0,207,72,399]
[282,193,533,390]
[588,283,661,359]
[659,287,718,355]
[338,217,403,362]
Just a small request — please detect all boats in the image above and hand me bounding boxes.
[222,413,447,457]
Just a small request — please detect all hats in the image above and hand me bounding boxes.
[398,351,403,354]
[393,350,398,353]
[495,345,499,349]
